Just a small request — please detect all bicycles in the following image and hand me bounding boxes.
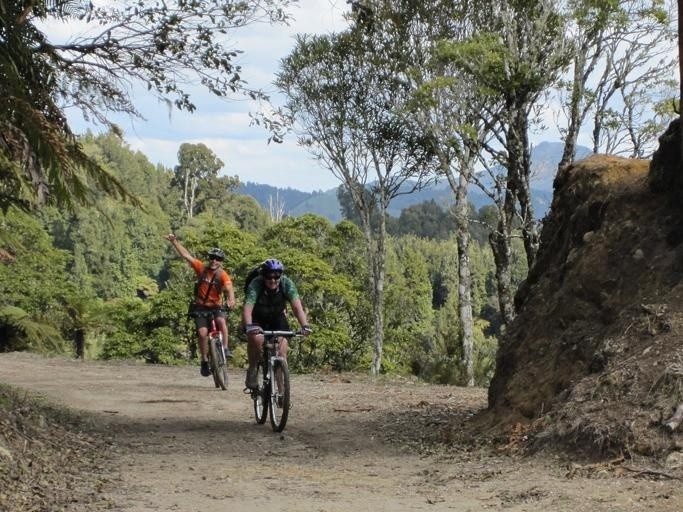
[185,307,234,391]
[243,328,311,432]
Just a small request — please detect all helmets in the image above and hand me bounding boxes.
[208,248,226,259]
[259,259,285,273]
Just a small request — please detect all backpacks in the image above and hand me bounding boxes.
[244,262,265,298]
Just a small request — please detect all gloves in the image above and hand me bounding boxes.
[301,325,313,336]
[244,323,265,336]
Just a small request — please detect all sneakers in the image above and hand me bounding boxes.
[200,357,210,377]
[278,396,294,410]
[246,367,259,390]
[224,349,234,357]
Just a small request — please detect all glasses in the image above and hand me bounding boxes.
[209,256,225,262]
[263,274,281,280]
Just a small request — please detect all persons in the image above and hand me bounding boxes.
[243,259,310,409]
[162,232,235,376]
